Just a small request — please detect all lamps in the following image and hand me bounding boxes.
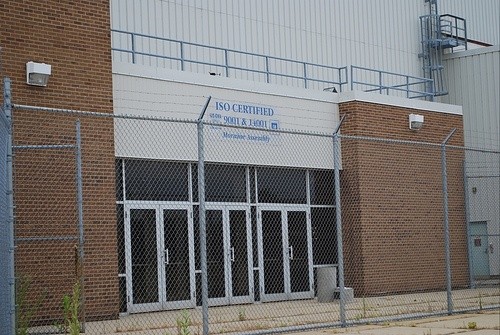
[24,60,51,88]
[409,112,425,131]
